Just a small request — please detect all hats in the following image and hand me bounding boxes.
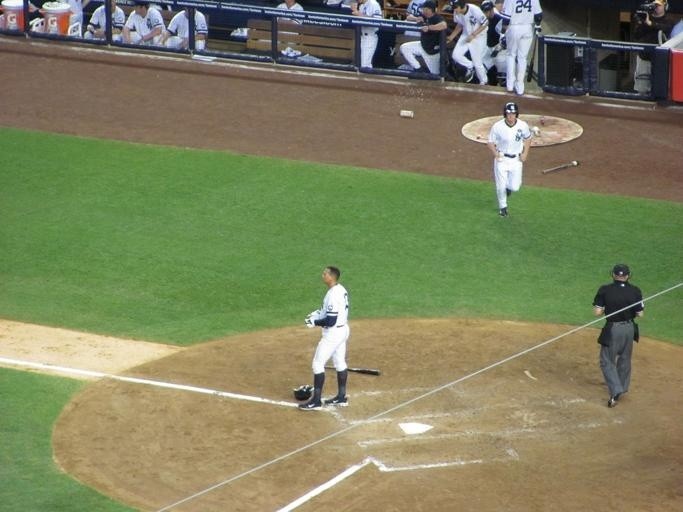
[418,1,436,8]
[613,264,631,278]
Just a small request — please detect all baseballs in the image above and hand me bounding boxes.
[532,126,539,131]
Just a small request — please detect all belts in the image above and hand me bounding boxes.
[503,153,519,158]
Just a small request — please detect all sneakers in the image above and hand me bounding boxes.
[299,400,322,410]
[609,392,623,407]
[463,66,474,83]
[325,397,348,406]
[500,209,508,217]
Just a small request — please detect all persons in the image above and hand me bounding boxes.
[295,263,352,413]
[0,0,682,97]
[591,260,645,411]
[486,97,533,219]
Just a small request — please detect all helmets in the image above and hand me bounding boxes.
[505,103,517,117]
[481,0,494,12]
[294,385,314,400]
[453,0,465,9]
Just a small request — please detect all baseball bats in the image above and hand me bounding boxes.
[542,162,579,174]
[325,368,380,376]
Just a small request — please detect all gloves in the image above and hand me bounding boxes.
[304,309,319,327]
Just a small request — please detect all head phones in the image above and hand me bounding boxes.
[665,0,669,11]
